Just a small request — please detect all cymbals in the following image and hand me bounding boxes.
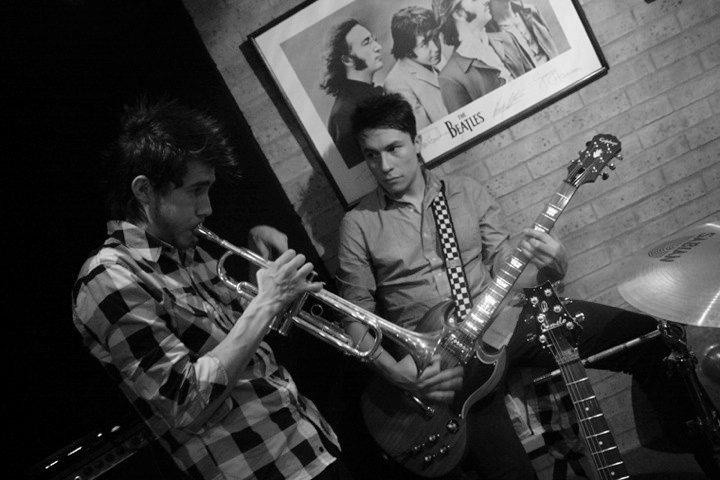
[617,220,720,330]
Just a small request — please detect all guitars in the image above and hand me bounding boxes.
[512,280,631,480]
[361,132,624,475]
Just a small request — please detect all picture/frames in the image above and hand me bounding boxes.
[246,0,609,217]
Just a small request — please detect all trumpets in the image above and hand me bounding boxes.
[195,222,446,380]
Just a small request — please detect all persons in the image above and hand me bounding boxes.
[383,6,448,133]
[72,96,353,480]
[432,0,536,114]
[320,18,386,169]
[484,0,560,69]
[338,94,720,480]
